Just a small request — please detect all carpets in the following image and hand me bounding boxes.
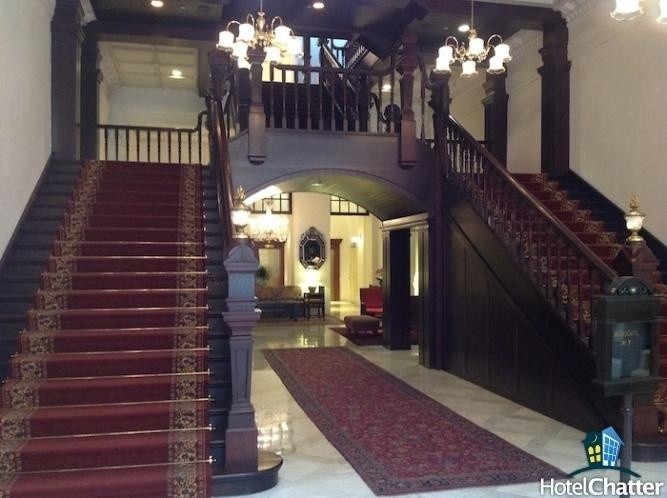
[328,327,385,346]
[260,347,574,495]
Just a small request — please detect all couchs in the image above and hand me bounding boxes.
[255,283,301,321]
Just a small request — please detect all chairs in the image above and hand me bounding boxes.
[359,287,384,318]
[304,286,325,320]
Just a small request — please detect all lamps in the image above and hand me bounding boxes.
[432,2,514,79]
[214,0,297,72]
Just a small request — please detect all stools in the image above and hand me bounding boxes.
[344,315,379,337]
